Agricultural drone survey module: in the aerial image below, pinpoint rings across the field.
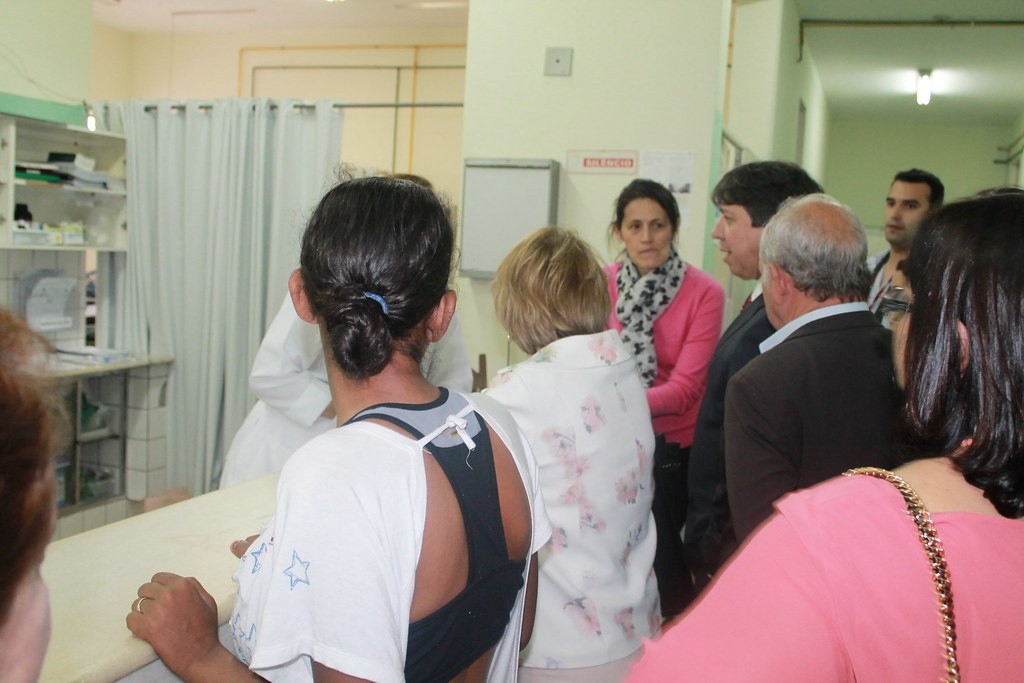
[137,598,145,612]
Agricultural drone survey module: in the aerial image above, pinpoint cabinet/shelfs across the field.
[0,112,129,251]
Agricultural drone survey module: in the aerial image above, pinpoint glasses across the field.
[880,286,914,330]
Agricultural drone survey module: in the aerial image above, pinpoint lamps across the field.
[915,67,932,105]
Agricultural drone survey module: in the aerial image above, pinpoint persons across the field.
[482,226,663,683]
[221,175,473,488]
[127,176,552,683]
[0,311,63,683]
[722,195,934,543]
[623,190,1024,683]
[599,178,726,609]
[683,161,826,597]
[866,168,945,390]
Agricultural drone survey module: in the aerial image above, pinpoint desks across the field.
[27,345,177,504]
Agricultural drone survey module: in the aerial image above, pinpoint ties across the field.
[739,291,752,314]
